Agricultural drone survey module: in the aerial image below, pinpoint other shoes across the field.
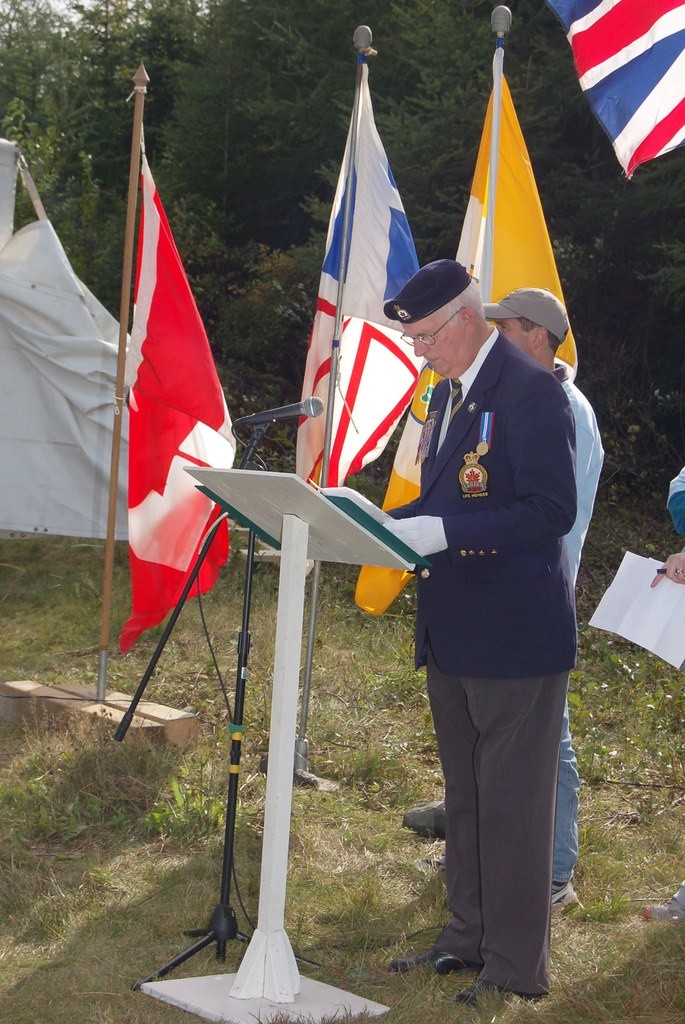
[551,886,577,913]
[647,904,672,918]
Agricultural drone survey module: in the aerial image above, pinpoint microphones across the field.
[231,396,324,428]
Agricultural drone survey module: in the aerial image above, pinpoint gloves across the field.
[383,515,448,557]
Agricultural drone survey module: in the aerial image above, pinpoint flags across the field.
[355,47,576,618]
[295,71,429,487]
[121,156,236,652]
[543,0,685,178]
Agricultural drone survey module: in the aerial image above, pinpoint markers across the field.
[656,569,678,574]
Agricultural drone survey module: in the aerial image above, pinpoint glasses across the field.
[400,307,465,346]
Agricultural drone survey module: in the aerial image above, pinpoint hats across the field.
[383,259,473,323]
[483,287,569,344]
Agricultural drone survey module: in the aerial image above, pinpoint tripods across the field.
[114,425,325,993]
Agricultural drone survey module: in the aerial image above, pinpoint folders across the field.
[195,484,433,568]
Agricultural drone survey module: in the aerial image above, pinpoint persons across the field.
[641,463,685,922]
[403,288,605,914]
[379,258,576,1007]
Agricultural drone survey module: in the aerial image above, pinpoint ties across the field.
[449,378,462,424]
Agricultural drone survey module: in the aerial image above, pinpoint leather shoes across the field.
[389,950,467,976]
[456,980,539,1006]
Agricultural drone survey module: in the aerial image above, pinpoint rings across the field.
[677,569,683,574]
